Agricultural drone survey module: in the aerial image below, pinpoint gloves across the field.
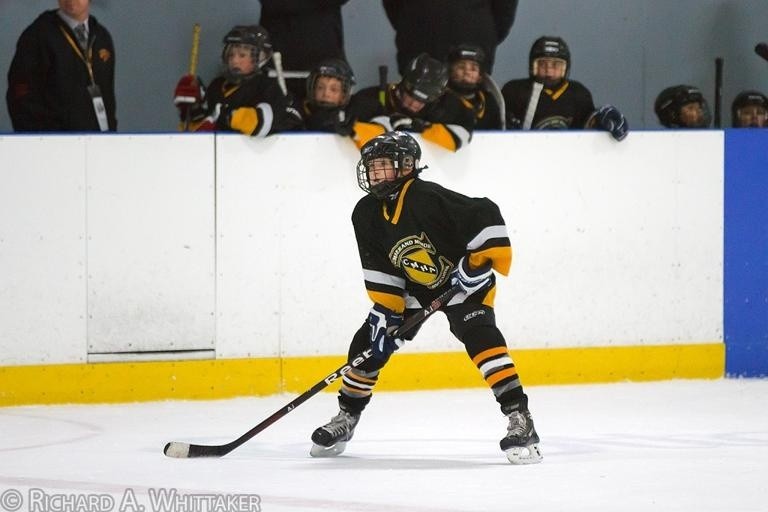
[366,302,403,361]
[175,74,233,128]
[595,106,628,141]
[450,256,492,295]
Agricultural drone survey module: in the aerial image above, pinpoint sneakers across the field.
[312,408,360,447]
[500,410,539,450]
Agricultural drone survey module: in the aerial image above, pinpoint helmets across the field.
[222,26,274,71]
[392,40,483,113]
[305,59,352,106]
[530,36,570,85]
[357,130,419,198]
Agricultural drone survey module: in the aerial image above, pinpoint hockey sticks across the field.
[165,288,463,458]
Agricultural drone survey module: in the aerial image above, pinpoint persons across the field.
[501,36,628,142]
[444,35,509,130]
[6,0,117,134]
[350,53,477,153]
[297,57,387,150]
[175,24,304,139]
[310,131,543,463]
